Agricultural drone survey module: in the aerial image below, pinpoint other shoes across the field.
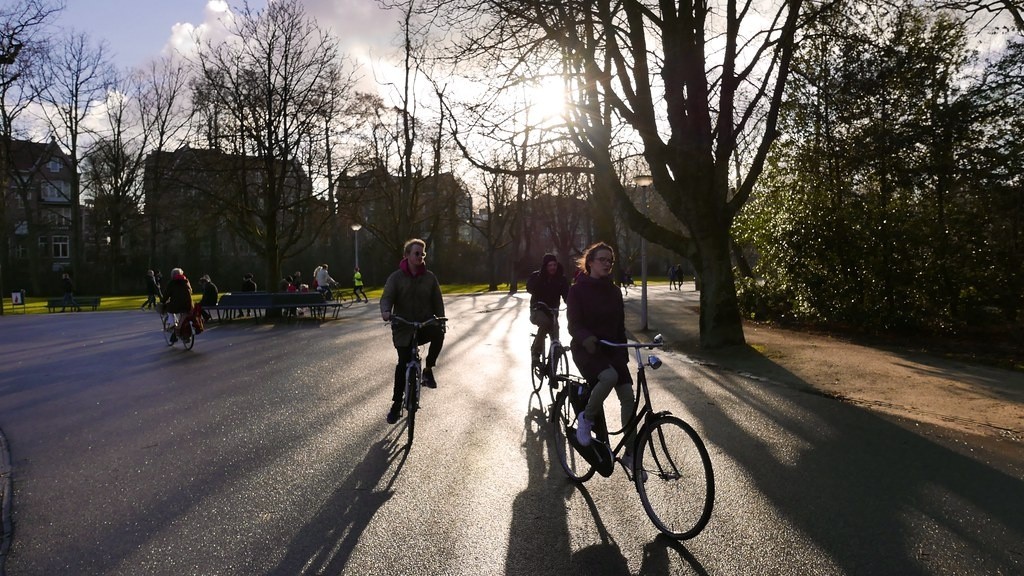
[169,323,175,329]
[532,355,540,363]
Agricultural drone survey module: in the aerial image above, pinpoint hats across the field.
[542,253,557,265]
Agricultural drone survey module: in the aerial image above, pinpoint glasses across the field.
[592,257,615,267]
[415,252,425,257]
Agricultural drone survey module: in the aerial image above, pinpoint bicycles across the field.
[551,333,715,540]
[384,314,448,444]
[530,302,571,405]
[315,282,353,308]
[155,300,195,350]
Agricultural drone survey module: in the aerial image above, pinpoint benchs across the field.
[44,296,100,313]
[202,291,343,325]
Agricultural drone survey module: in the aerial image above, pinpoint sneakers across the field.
[422,368,436,388]
[622,452,648,482]
[577,411,591,446]
[387,404,401,423]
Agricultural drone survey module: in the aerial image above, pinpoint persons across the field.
[160,268,192,343]
[312,264,338,301]
[526,253,572,389]
[620,266,637,289]
[380,240,445,424]
[236,273,256,318]
[198,275,218,322]
[353,267,369,304]
[573,267,580,283]
[141,269,161,311]
[60,273,82,312]
[567,244,648,484]
[669,264,684,291]
[279,271,309,316]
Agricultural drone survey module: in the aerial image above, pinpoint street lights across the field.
[634,175,654,330]
[351,224,362,267]
